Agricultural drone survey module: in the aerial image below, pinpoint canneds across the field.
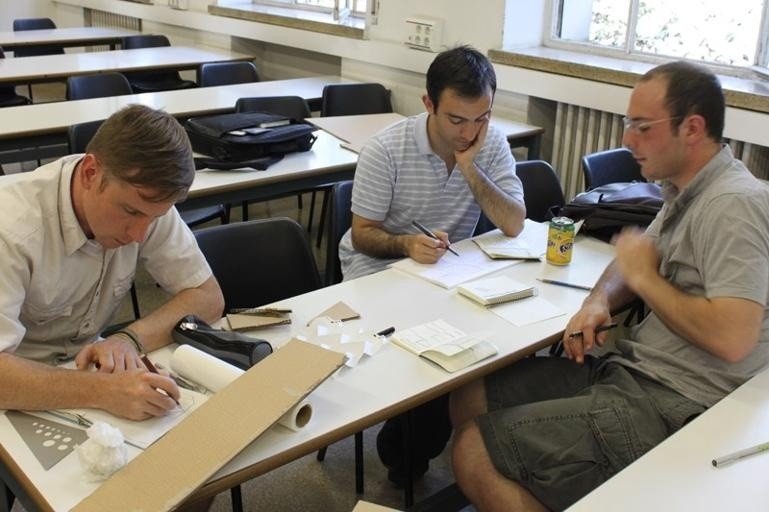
[545,216,575,265]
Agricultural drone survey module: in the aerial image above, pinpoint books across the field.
[392,319,497,373]
[458,274,536,306]
[388,240,526,290]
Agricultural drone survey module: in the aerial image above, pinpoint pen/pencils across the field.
[712,441,769,467]
[373,326,395,338]
[411,219,459,257]
[139,353,182,409]
[568,323,618,337]
[536,278,593,292]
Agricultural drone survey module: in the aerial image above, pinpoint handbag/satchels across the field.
[546,183,663,230]
[171,316,272,367]
[185,113,316,170]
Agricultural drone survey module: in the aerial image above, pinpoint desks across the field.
[562,362,769,512]
[0,219,636,512]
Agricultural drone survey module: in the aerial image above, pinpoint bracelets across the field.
[106,330,142,353]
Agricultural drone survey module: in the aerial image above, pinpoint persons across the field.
[449,60,769,511]
[0,102,226,512]
[337,42,526,488]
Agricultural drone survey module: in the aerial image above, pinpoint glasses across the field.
[623,117,677,134]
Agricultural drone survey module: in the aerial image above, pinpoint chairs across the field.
[480,161,572,356]
[584,149,644,193]
[331,178,365,285]
[190,218,365,511]
[0,16,547,241]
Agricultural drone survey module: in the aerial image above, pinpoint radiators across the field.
[90,10,140,55]
[546,100,769,205]
